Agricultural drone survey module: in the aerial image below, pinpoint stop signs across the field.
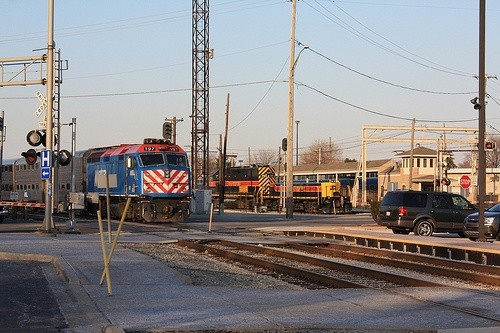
[460,176,472,189]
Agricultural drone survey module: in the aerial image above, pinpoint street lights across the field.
[294,120,301,167]
[284,45,311,218]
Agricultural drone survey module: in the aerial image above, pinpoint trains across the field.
[0,142,196,223]
[192,164,353,214]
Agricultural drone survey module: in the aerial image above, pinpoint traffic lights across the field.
[432,179,440,186]
[485,141,494,149]
[20,149,39,164]
[440,179,450,185]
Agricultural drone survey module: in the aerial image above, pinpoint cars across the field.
[461,202,500,242]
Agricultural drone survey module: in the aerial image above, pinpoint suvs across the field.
[376,188,479,237]
